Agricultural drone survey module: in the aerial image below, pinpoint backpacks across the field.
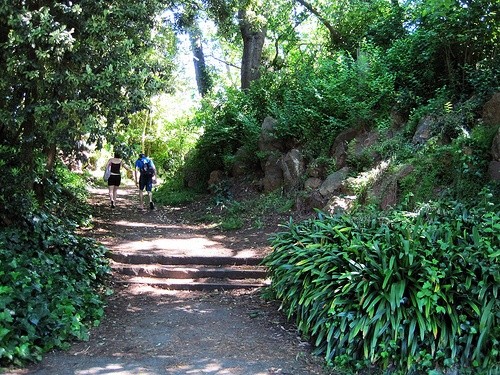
[139,158,154,177]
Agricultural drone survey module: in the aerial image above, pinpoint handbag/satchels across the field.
[104,158,112,182]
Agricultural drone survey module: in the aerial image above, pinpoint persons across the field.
[134,154,157,209]
[106,151,132,208]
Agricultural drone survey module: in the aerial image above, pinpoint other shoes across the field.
[111,201,115,208]
[150,202,154,209]
